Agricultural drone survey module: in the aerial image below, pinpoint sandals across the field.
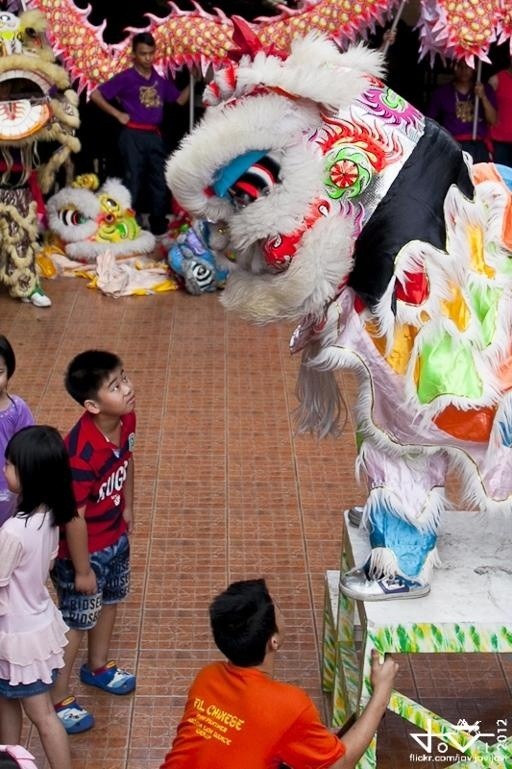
[77,658,138,696]
[54,694,95,736]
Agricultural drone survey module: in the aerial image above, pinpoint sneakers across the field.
[347,505,368,528]
[337,562,433,602]
[19,287,53,307]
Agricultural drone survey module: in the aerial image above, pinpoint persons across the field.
[43,349,139,737]
[154,577,401,769]
[1,333,36,528]
[0,79,53,309]
[350,24,398,55]
[429,54,497,165]
[1,422,83,768]
[484,51,512,167]
[331,438,447,602]
[90,31,213,240]
[0,740,38,768]
